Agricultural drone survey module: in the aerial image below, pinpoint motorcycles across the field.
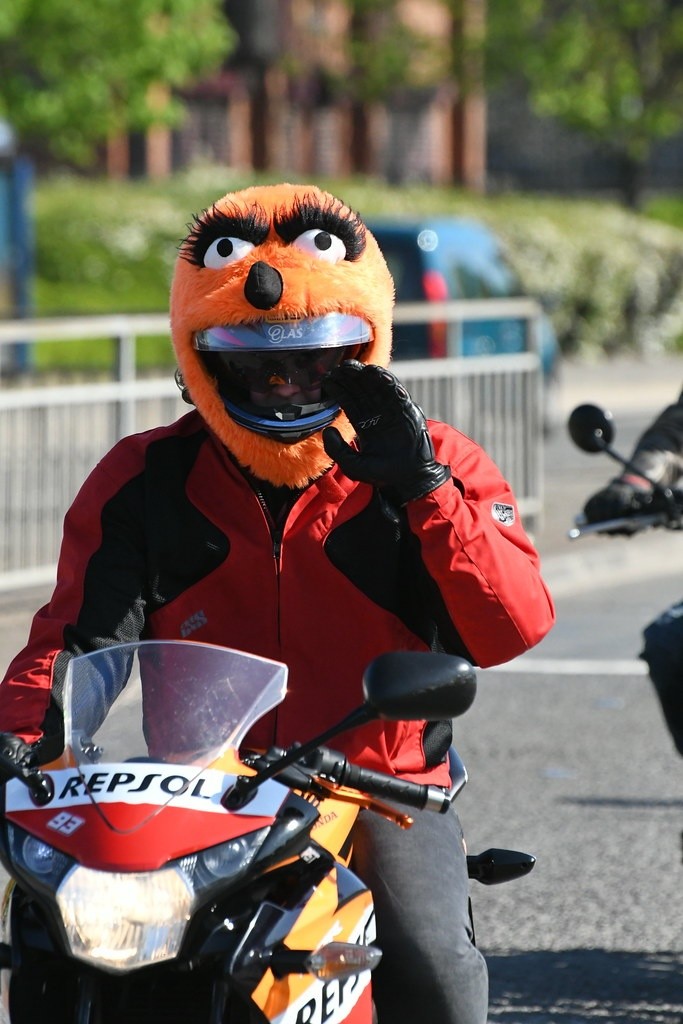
[0,640,537,1024]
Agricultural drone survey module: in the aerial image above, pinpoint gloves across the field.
[583,478,657,538]
[323,360,451,510]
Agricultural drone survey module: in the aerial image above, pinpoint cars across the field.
[362,215,558,382]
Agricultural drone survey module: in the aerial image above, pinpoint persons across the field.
[1,182,558,1023]
[580,382,683,754]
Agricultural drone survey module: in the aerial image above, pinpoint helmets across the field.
[168,185,397,493]
